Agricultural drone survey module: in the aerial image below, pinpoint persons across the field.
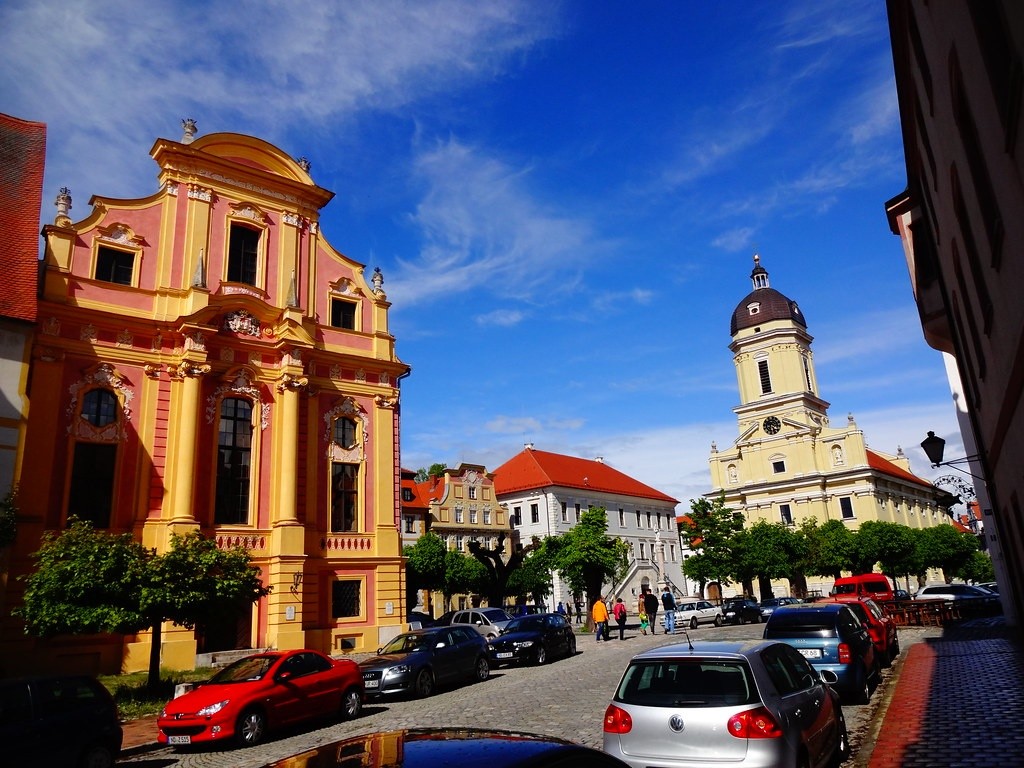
[557,601,583,624]
[592,587,678,643]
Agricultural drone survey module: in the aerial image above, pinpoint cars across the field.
[659,601,723,630]
[488,613,576,666]
[719,597,762,625]
[257,726,634,768]
[762,604,884,706]
[157,649,365,747]
[814,595,902,662]
[602,639,850,768]
[0,673,123,768]
[357,624,490,700]
[892,581,999,609]
[407,605,548,658]
[759,597,801,623]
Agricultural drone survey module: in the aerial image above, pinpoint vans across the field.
[830,574,895,604]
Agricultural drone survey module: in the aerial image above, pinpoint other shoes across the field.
[640,629,647,636]
[664,629,668,634]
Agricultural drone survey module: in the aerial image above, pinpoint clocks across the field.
[763,416,782,436]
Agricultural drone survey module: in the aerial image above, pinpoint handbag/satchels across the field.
[619,610,627,621]
[579,607,581,611]
[562,610,566,616]
[639,612,648,621]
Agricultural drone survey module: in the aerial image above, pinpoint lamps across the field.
[290,571,303,595]
[975,527,999,551]
[920,431,992,485]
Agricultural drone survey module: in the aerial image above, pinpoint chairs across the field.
[646,662,737,695]
